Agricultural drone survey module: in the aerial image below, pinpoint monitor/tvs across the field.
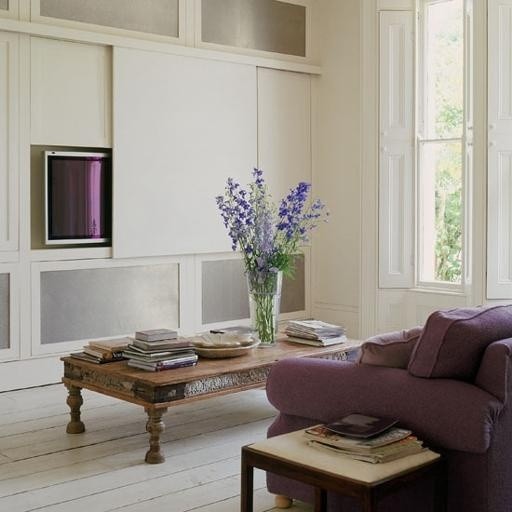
[45,151,112,245]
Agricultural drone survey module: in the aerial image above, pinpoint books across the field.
[210,327,259,335]
[302,413,429,464]
[70,329,198,372]
[280,320,347,347]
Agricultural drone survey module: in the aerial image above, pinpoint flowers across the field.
[214,163,331,341]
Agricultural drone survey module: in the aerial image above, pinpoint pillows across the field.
[356,326,423,369]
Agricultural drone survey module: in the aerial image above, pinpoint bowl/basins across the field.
[189,334,261,358]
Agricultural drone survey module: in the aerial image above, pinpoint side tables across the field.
[239,422,441,511]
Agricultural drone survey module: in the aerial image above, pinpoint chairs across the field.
[264,302,511,511]
